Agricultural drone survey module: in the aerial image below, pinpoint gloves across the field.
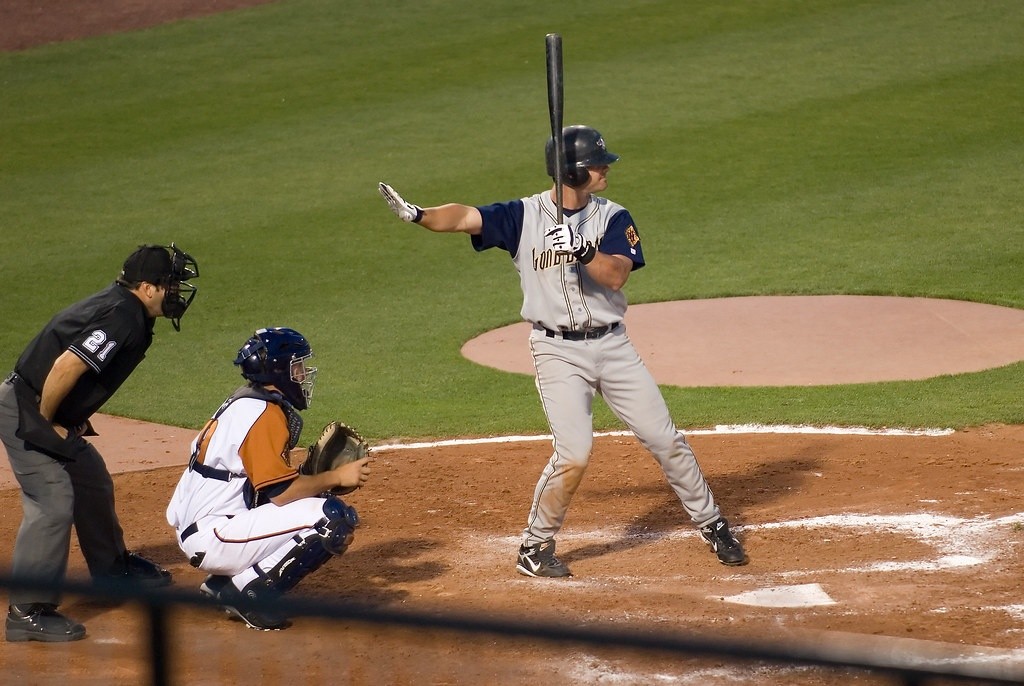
[544,224,596,266]
[190,551,205,568]
[379,182,424,223]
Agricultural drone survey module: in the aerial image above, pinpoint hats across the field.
[121,246,192,283]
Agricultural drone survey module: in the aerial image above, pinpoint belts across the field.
[543,322,619,341]
[181,515,234,543]
[7,372,18,384]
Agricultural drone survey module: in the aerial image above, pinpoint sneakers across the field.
[200,573,231,597]
[699,517,746,565]
[92,553,172,586]
[5,602,86,642]
[217,580,287,631]
[516,539,573,577]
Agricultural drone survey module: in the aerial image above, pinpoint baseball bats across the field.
[545,32,568,256]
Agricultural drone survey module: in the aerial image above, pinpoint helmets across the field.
[545,125,619,186]
[234,328,317,411]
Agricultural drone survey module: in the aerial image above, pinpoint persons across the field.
[377,124,745,578]
[0,243,201,642]
[165,326,372,631]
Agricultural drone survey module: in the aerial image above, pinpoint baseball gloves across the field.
[298,421,371,496]
